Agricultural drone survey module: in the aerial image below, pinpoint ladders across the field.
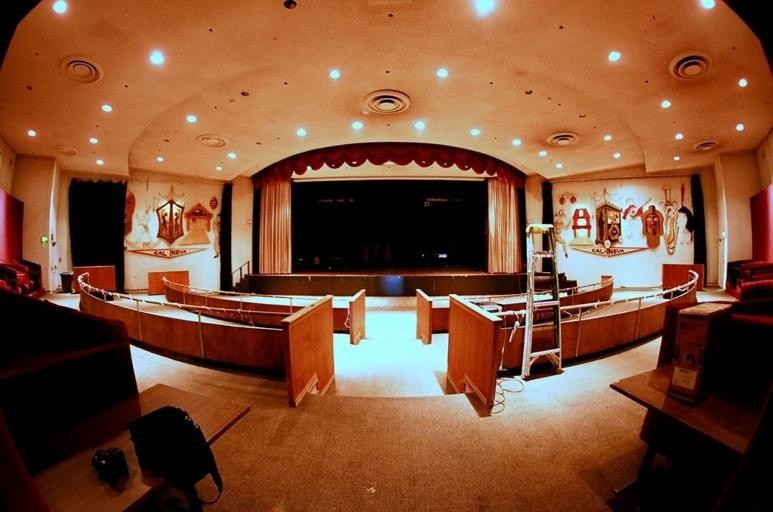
[521,224,565,381]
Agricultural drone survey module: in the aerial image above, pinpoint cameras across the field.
[90,447,128,474]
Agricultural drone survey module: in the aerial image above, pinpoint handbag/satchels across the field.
[127,405,223,505]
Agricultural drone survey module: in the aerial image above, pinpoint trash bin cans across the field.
[59,272,74,293]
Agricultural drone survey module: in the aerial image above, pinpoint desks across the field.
[604,367,765,512]
[5,385,251,512]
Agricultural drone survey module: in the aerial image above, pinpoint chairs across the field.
[0,256,46,297]
[722,259,773,302]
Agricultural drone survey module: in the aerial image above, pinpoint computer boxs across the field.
[665,302,733,405]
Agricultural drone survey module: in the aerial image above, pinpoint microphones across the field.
[508,320,521,343]
